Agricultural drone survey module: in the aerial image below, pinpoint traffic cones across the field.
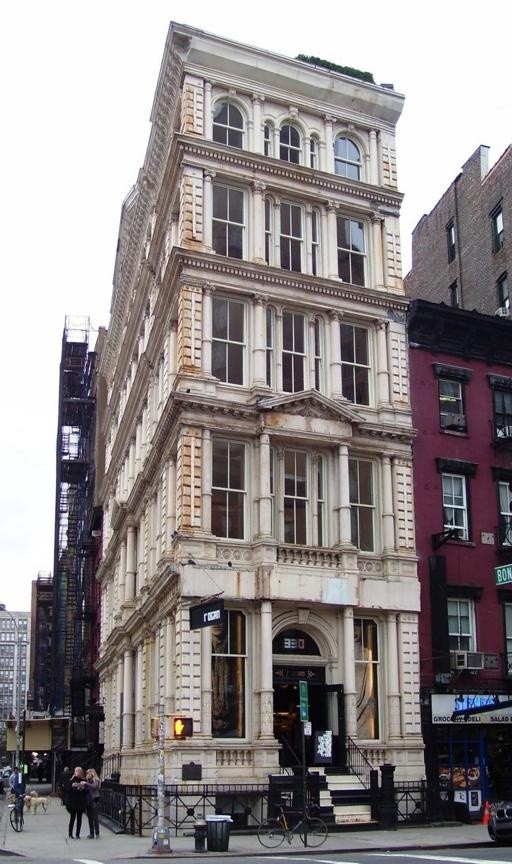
[481,799,490,826]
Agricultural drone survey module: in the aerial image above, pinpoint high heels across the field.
[69,832,80,838]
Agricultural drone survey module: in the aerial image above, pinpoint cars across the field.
[488,796,511,847]
[0,767,13,788]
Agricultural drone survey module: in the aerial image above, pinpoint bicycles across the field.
[257,800,329,849]
[10,792,27,832]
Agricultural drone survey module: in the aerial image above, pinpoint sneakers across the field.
[87,834,101,839]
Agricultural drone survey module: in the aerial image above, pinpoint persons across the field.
[80,768,101,839]
[65,766,87,840]
[8,766,25,802]
[36,763,44,783]
[58,766,70,806]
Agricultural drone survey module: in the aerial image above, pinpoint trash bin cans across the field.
[205,815,233,852]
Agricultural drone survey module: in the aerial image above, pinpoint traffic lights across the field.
[174,717,193,737]
